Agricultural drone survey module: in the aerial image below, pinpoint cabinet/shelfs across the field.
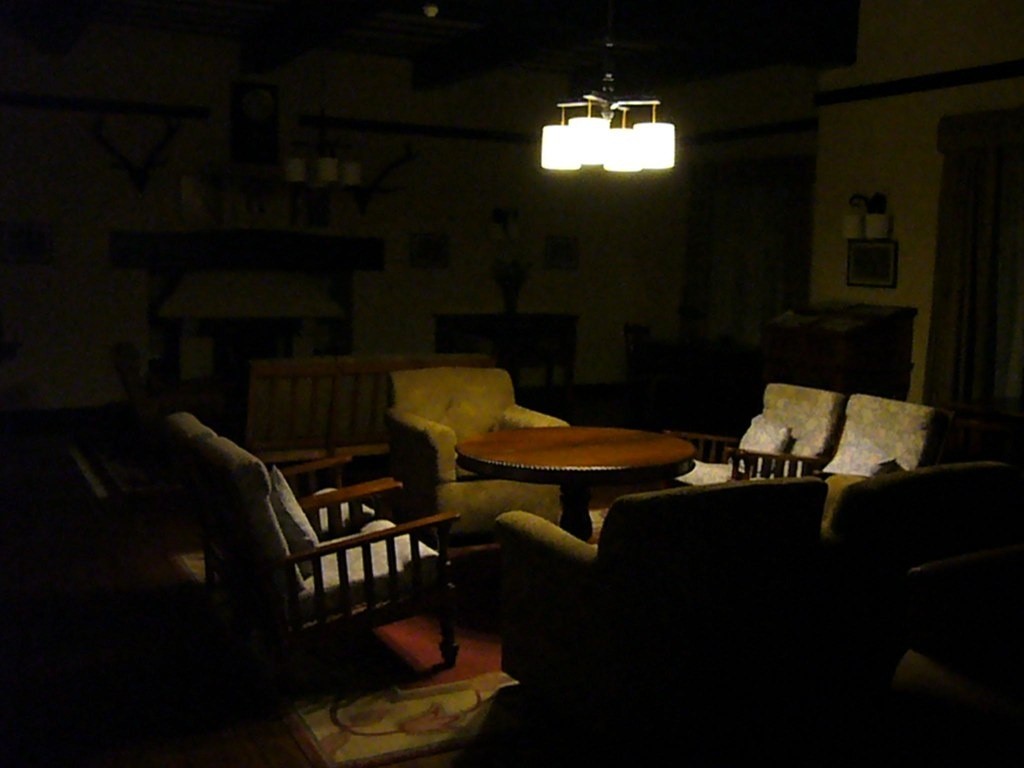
[436,312,579,421]
[762,301,919,401]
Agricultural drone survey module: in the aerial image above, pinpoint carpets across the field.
[161,508,613,767]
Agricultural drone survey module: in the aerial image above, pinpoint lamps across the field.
[533,0,688,188]
[281,52,365,188]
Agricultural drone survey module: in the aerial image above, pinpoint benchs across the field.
[241,354,496,491]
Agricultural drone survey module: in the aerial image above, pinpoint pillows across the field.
[728,415,791,473]
[822,434,894,478]
[269,464,319,576]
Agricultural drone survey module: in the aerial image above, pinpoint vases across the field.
[495,263,526,311]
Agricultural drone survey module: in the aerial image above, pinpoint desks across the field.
[453,425,697,543]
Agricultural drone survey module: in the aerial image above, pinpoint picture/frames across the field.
[845,236,899,289]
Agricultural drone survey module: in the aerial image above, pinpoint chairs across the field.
[165,411,462,719]
[662,381,955,487]
[113,343,227,433]
[384,365,570,555]
[494,458,1024,768]
